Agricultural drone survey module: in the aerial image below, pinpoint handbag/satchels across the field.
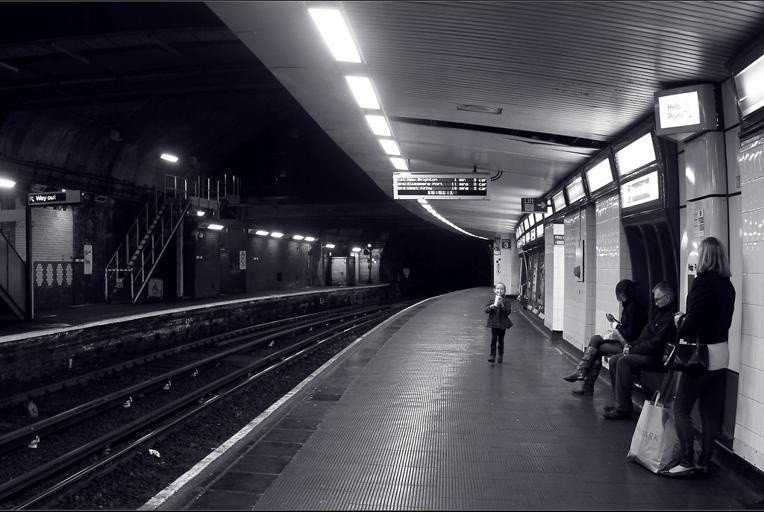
[663,313,709,372]
[626,390,682,475]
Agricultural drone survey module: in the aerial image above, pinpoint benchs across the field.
[603,312,687,406]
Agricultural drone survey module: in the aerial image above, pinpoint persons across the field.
[484,281,514,365]
[601,280,679,420]
[658,237,736,478]
[563,279,650,399]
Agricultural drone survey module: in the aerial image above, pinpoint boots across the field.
[564,346,602,395]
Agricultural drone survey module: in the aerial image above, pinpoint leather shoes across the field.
[659,469,696,476]
[603,404,634,419]
